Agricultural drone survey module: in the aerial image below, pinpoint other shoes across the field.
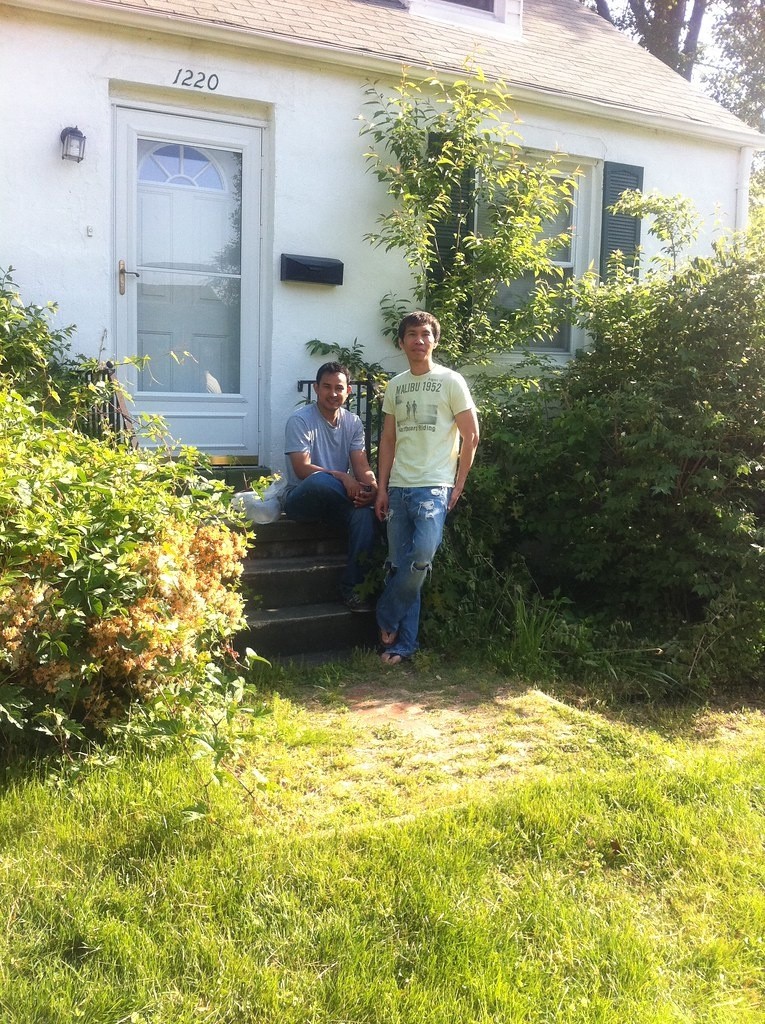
[349,591,373,612]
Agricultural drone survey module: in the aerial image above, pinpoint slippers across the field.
[381,653,404,665]
[379,625,396,647]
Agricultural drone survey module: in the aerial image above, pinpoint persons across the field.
[373,311,479,666]
[283,362,386,613]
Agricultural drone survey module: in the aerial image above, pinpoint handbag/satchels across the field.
[231,472,288,524]
